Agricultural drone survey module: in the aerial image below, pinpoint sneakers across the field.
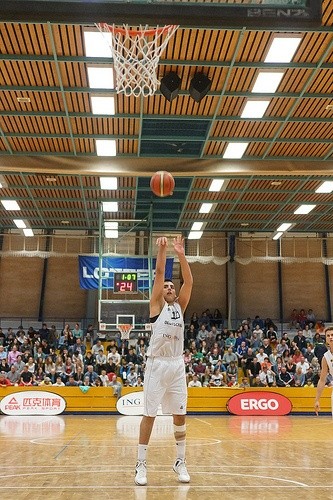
[173,458,190,482]
[135,460,147,486]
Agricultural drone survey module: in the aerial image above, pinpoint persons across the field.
[314,326,333,419]
[0,322,148,388]
[185,308,333,387]
[134,235,193,486]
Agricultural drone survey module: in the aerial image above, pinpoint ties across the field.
[90,372,92,384]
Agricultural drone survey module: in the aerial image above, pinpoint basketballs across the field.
[150,171,176,198]
